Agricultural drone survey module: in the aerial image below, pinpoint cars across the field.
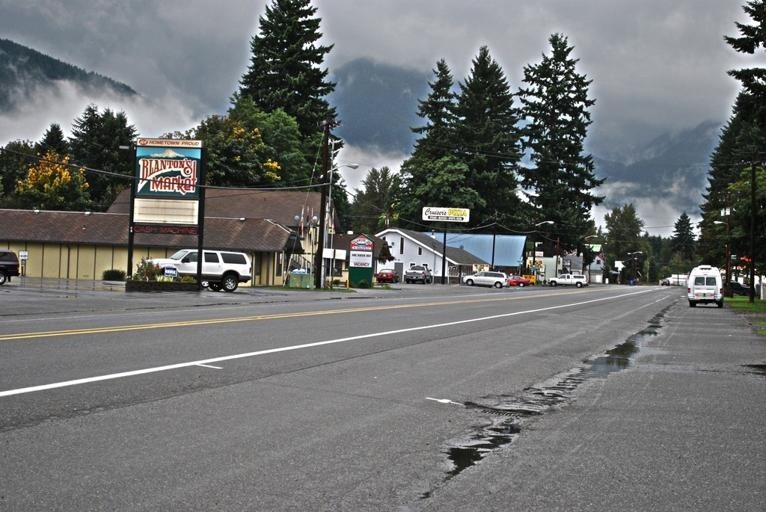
[0,251,20,285]
[463,271,530,288]
[140,249,252,292]
[661,280,670,286]
[376,269,400,282]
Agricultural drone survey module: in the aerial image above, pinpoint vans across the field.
[688,265,723,307]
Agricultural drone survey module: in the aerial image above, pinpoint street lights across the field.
[714,221,730,286]
[588,235,598,284]
[533,221,554,275]
[314,118,359,288]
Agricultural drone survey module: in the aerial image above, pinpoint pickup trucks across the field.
[548,274,587,288]
[405,266,432,284]
